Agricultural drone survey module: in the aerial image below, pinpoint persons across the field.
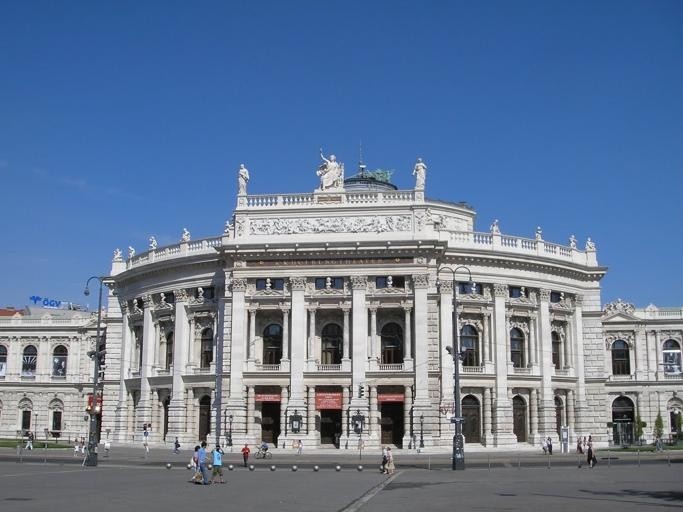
[186,442,225,484]
[295,440,302,455]
[173,436,180,455]
[318,148,343,187]
[542,435,597,468]
[238,162,248,196]
[240,442,250,468]
[411,157,427,189]
[24,432,33,450]
[80,436,87,456]
[385,447,394,474]
[72,438,79,456]
[256,441,268,457]
[380,447,388,475]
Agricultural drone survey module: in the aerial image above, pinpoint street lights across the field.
[433,264,477,470]
[417,413,423,449]
[83,274,121,468]
[227,413,233,446]
[33,411,38,438]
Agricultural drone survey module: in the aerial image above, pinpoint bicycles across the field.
[255,446,272,460]
